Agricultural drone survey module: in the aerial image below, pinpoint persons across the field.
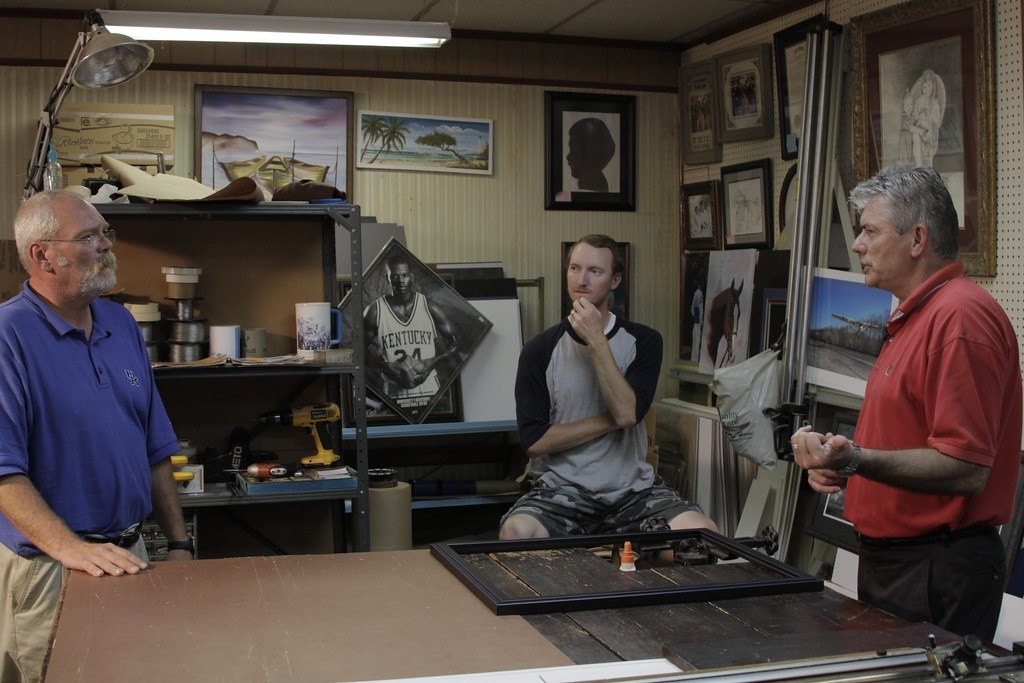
[690,278,704,365]
[690,74,757,131]
[790,160,1024,645]
[499,235,719,560]
[0,188,192,683]
[692,200,710,234]
[362,256,458,419]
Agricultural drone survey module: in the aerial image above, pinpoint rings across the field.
[792,444,798,449]
[570,311,576,317]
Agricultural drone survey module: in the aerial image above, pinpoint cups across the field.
[245,328,267,357]
[296,302,342,361]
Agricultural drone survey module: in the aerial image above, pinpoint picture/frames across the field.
[543,89,638,211]
[797,266,901,399]
[848,0,997,280]
[678,11,826,165]
[338,236,493,424]
[357,109,494,175]
[558,239,630,324]
[807,407,866,557]
[678,157,792,375]
[193,83,354,203]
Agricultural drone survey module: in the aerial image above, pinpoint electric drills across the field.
[247,403,340,466]
[222,463,292,478]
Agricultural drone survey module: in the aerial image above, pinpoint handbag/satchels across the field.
[707,322,787,473]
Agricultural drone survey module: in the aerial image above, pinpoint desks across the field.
[39,540,1024,683]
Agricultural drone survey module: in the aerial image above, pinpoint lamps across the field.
[97,7,451,48]
[21,10,155,206]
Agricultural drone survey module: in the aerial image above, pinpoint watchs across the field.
[834,444,861,477]
[167,537,196,554]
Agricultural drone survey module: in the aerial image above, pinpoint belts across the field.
[82,519,145,548]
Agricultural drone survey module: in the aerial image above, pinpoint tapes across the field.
[168,282,196,299]
[166,274,198,283]
[124,302,158,313]
[131,312,161,321]
[161,265,202,274]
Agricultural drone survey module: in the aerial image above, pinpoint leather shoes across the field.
[854,519,999,549]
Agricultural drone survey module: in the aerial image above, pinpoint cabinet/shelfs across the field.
[21,206,372,556]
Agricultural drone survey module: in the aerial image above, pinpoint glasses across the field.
[40,229,117,249]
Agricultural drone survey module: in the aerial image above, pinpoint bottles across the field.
[619,541,636,571]
